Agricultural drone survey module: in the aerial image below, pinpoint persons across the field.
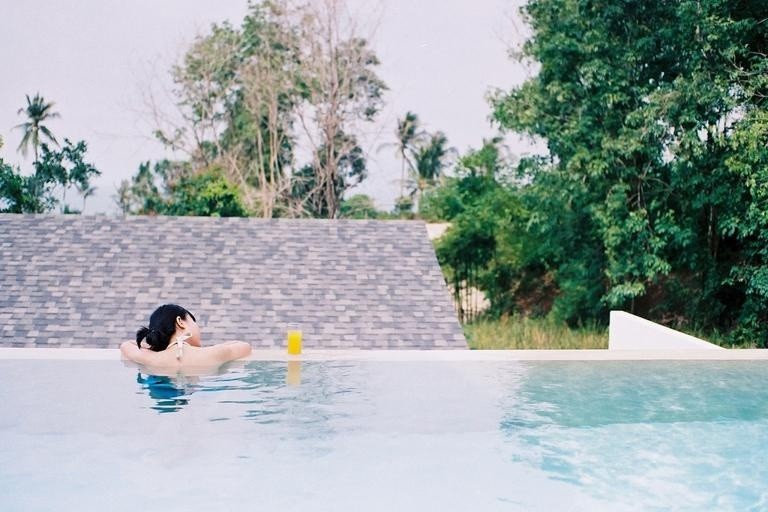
[121,303,251,379]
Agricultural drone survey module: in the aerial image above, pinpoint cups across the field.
[286,322,303,356]
